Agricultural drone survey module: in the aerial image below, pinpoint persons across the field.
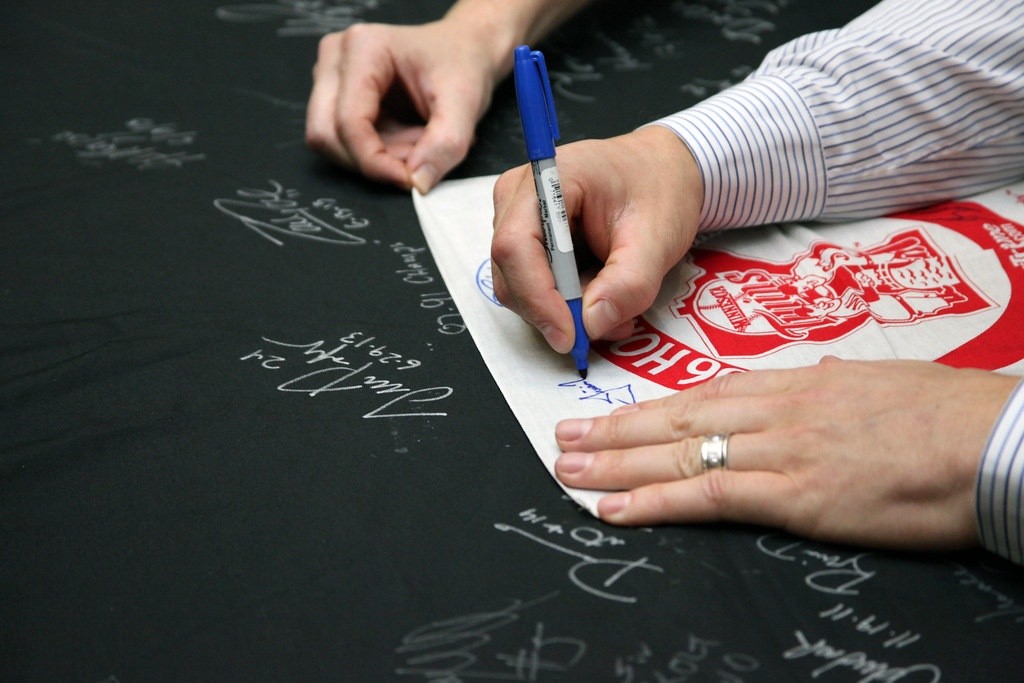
[305,0,585,195]
[491,0,1024,566]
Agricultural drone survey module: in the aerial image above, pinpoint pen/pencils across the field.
[514,44,592,381]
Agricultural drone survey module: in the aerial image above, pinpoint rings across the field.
[700,432,734,470]
